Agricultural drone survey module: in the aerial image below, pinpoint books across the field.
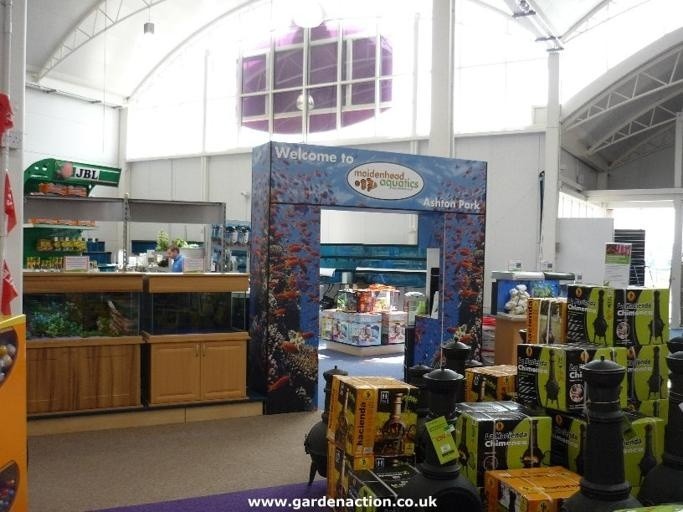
[485,465,583,512]
[328,443,415,512]
[465,365,517,401]
[630,343,670,401]
[556,412,664,483]
[454,401,552,488]
[348,468,423,510]
[526,296,568,346]
[568,286,672,345]
[516,347,628,410]
[327,374,420,456]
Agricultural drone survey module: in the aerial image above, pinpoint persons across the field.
[168,245,184,272]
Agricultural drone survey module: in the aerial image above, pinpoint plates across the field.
[146,272,250,407]
[23,270,144,418]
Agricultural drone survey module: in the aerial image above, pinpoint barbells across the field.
[273,308,287,317]
[267,375,290,394]
[447,326,458,334]
[274,291,298,300]
[458,289,478,297]
[287,244,301,253]
[302,332,313,338]
[278,341,299,356]
[461,262,480,273]
[285,260,298,267]
[459,335,474,343]
